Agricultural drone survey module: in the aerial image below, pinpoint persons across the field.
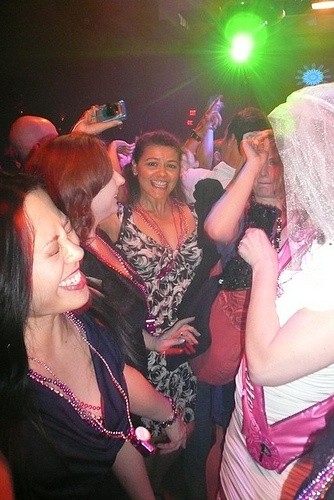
[1,171,188,500]
[92,130,205,443]
[189,104,287,487]
[3,102,60,167]
[199,105,272,171]
[17,133,203,455]
[216,81,333,500]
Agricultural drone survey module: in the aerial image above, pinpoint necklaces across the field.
[135,203,191,251]
[90,233,150,291]
[23,310,158,453]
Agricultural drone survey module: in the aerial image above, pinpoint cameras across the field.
[94,98,127,123]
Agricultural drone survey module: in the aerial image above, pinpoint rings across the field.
[180,331,183,336]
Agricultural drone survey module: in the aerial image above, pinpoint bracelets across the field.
[157,399,183,430]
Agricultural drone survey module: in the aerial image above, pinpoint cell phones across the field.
[135,441,157,456]
